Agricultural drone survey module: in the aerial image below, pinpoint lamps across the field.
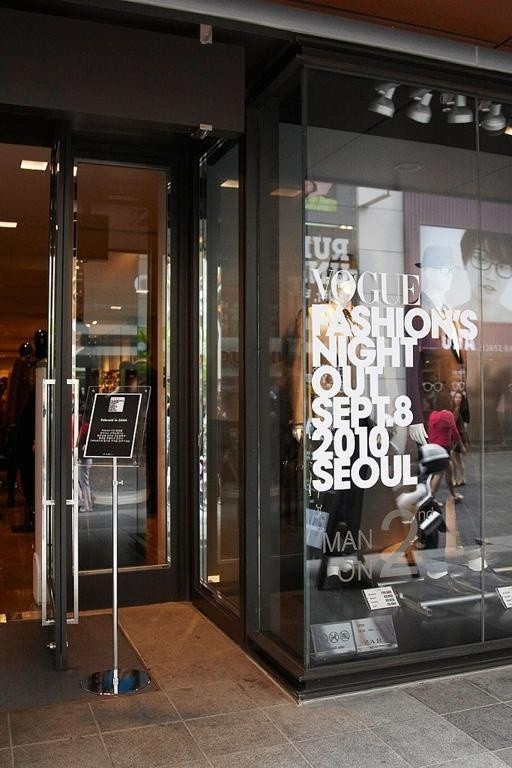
[366,80,507,137]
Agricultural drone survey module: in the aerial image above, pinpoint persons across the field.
[290,253,375,591]
[77,458,92,512]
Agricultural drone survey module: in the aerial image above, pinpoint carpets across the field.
[0,612,160,722]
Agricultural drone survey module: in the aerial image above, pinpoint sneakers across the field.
[434,476,467,507]
